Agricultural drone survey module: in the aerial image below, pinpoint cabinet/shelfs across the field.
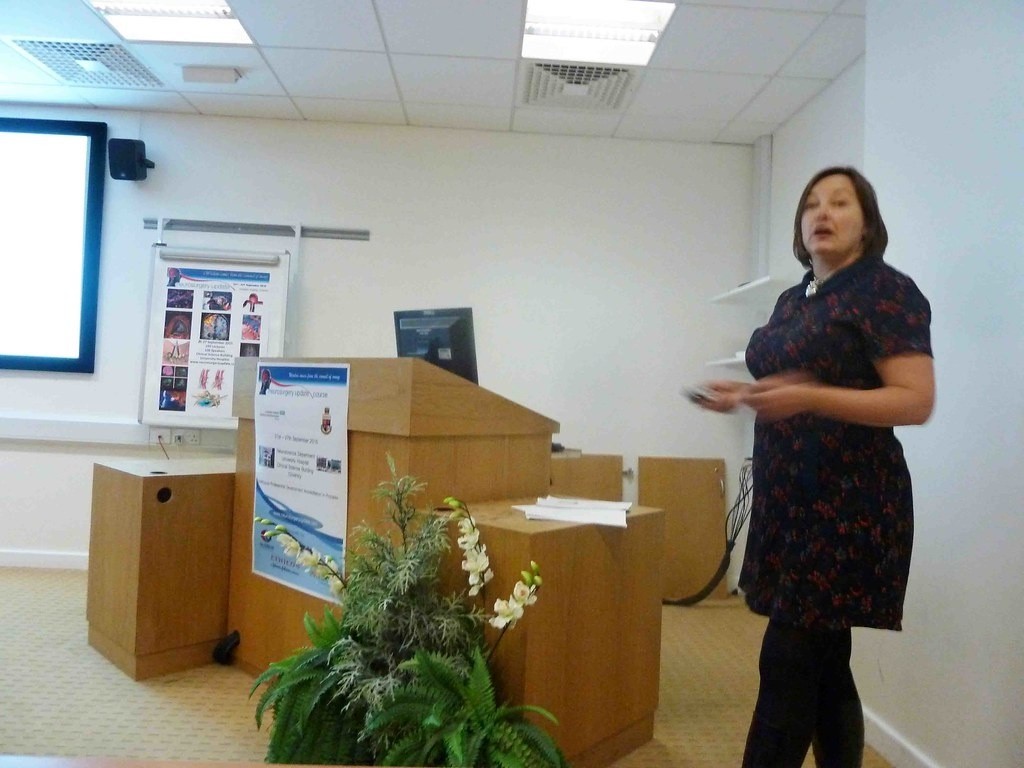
[707,276,786,373]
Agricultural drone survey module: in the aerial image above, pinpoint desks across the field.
[413,493,664,768]
[86,459,237,682]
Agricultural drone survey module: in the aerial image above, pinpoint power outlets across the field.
[149,427,171,445]
[172,429,200,445]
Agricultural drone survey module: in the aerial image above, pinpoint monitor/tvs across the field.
[394,306,480,388]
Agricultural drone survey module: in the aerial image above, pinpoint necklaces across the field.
[804,259,845,296]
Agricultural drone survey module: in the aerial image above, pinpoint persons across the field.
[696,166,936,768]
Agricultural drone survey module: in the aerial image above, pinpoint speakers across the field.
[108,139,148,181]
